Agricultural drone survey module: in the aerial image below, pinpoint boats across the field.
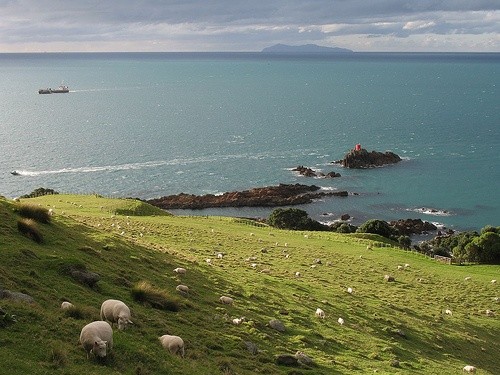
[38,87,69,94]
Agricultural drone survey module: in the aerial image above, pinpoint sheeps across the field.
[38,198,500,375]
